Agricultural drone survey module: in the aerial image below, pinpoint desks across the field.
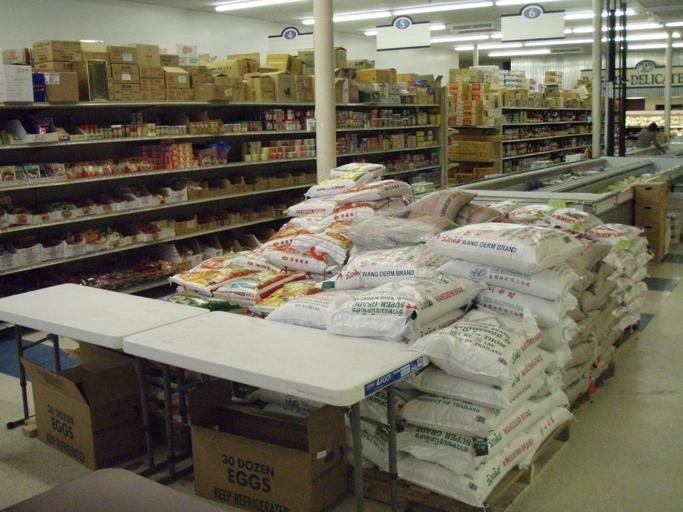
[1,466,244,510]
[0,281,210,485]
[123,308,427,507]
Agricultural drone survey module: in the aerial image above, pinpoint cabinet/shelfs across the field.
[0,99,619,295]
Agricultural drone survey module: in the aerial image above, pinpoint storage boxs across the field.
[0,40,398,104]
[181,381,353,511]
[15,343,143,471]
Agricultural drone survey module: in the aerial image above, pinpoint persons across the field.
[635,122,666,154]
